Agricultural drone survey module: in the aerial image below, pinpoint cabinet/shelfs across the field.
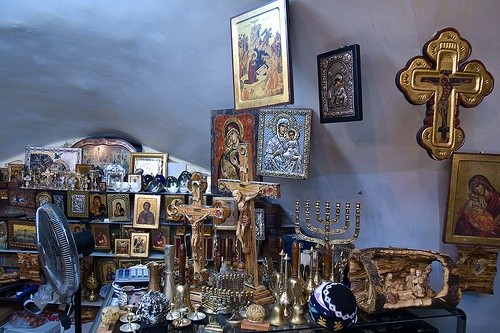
[0,189,276,309]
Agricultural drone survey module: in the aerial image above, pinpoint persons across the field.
[225,185,264,255]
[183,210,210,265]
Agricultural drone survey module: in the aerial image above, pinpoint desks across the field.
[88,279,467,333]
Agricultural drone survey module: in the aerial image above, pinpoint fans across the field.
[36,204,96,333]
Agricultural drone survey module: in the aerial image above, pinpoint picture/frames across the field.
[210,0,313,193]
[129,152,167,184]
[442,152,500,244]
[316,43,363,124]
[0,146,95,186]
[0,189,265,333]
